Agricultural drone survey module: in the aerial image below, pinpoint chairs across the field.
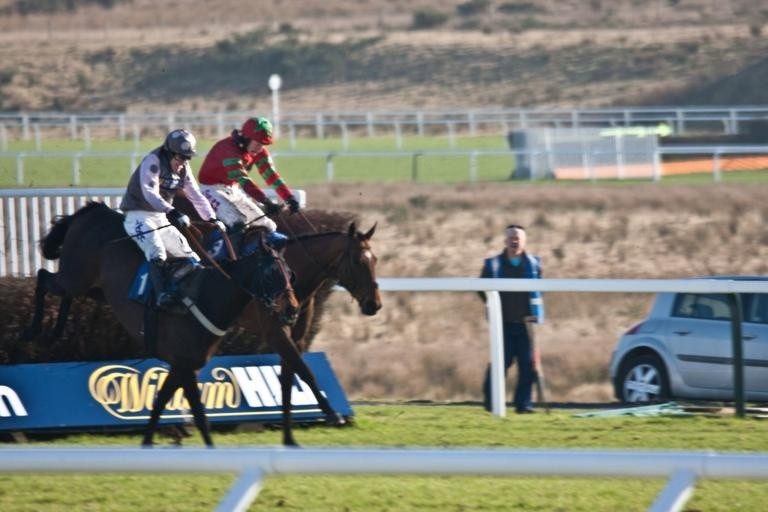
[688,303,714,319]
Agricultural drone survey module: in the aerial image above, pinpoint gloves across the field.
[263,197,281,216]
[168,210,190,228]
[210,219,227,232]
[287,197,299,211]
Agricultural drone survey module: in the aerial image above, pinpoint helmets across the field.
[242,117,273,145]
[163,129,198,157]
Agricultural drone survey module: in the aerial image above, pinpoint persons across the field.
[119,127,230,309]
[476,221,547,415]
[196,115,302,234]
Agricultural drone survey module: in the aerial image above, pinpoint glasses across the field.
[166,130,191,162]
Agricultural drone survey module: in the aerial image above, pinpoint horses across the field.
[18,200,303,447]
[189,222,382,446]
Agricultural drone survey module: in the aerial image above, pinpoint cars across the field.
[606,273,767,407]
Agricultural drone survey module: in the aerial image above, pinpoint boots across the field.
[150,258,173,312]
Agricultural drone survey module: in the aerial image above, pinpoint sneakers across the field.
[517,407,533,413]
